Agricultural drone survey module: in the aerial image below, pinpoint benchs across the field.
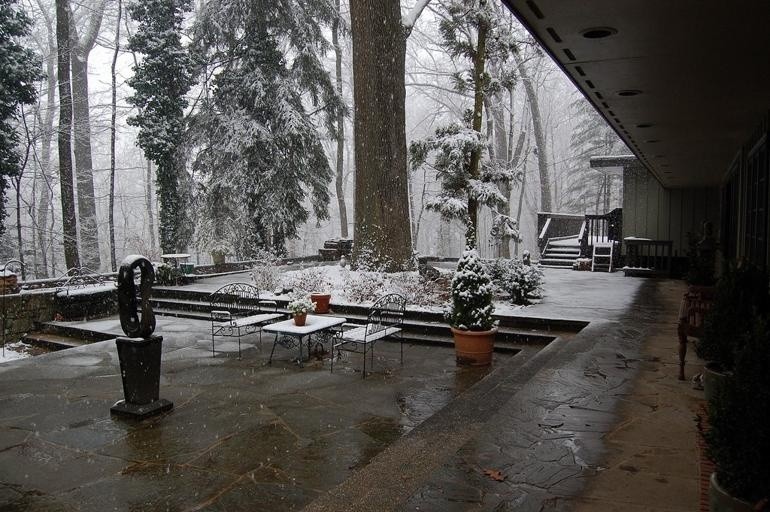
[678,285,717,381]
[330,292,408,380]
[208,281,285,361]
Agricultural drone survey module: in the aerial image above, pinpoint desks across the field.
[261,315,348,370]
[161,253,190,288]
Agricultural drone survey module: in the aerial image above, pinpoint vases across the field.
[311,292,331,314]
[441,244,500,367]
[294,314,306,326]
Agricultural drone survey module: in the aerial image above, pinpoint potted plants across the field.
[697,256,770,512]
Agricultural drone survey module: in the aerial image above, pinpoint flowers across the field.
[288,300,310,314]
